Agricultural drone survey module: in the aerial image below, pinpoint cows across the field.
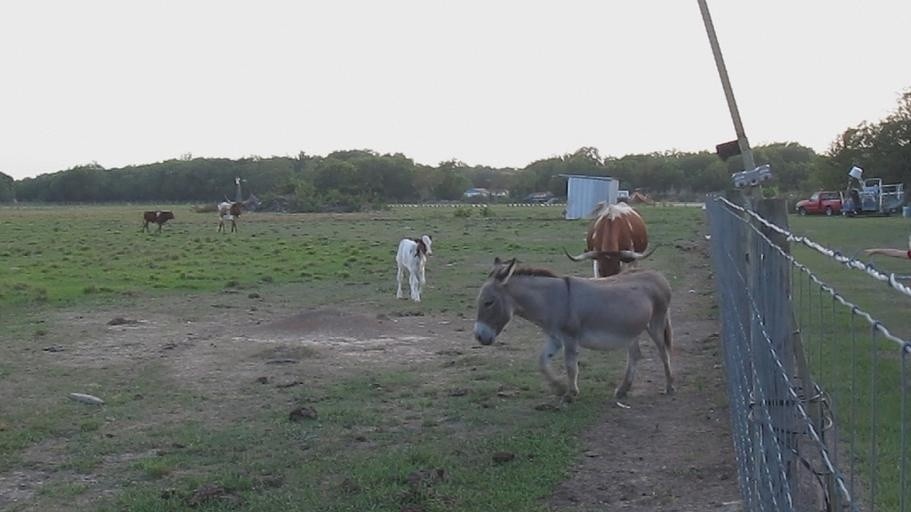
[216,201,242,234]
[141,210,175,233]
[561,201,663,279]
[395,235,433,303]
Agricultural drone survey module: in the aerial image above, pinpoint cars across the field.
[521,191,559,204]
[462,187,512,202]
[795,190,843,216]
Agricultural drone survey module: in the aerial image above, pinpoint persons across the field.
[216,199,231,233]
[863,235,911,260]
[224,193,241,233]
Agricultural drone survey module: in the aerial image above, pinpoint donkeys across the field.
[472,257,675,408]
